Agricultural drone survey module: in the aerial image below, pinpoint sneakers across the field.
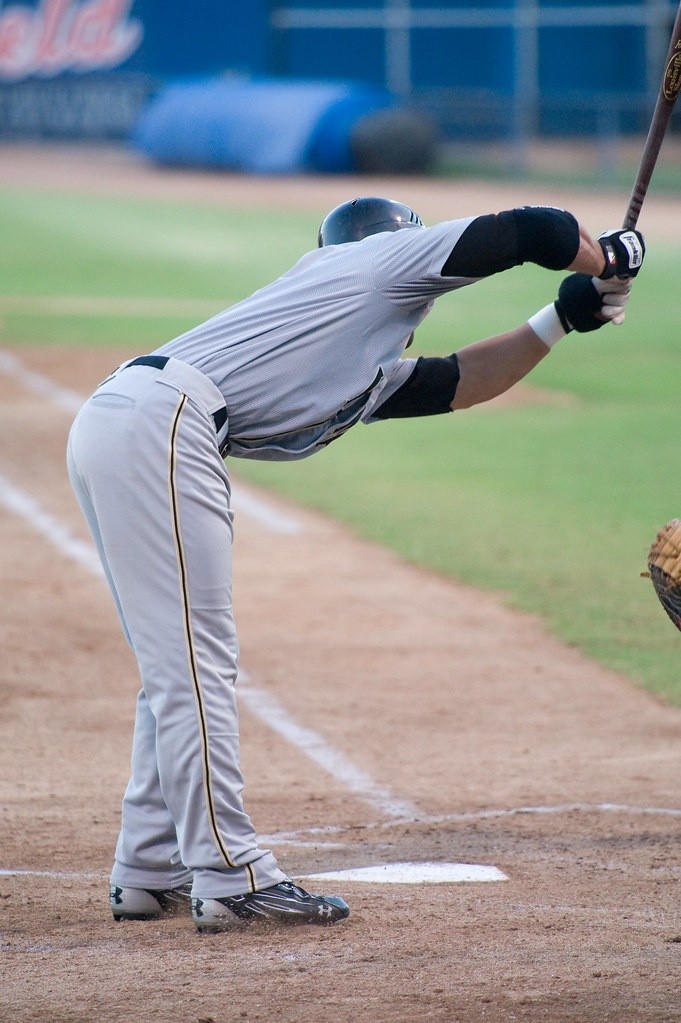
[110,882,352,933]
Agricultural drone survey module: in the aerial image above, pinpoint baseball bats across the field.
[618,0,681,232]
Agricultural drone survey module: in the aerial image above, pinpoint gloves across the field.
[559,227,647,333]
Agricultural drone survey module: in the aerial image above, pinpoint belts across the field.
[123,356,233,461]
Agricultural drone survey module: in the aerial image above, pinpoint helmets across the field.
[319,197,425,244]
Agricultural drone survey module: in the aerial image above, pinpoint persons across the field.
[67,197,646,932]
